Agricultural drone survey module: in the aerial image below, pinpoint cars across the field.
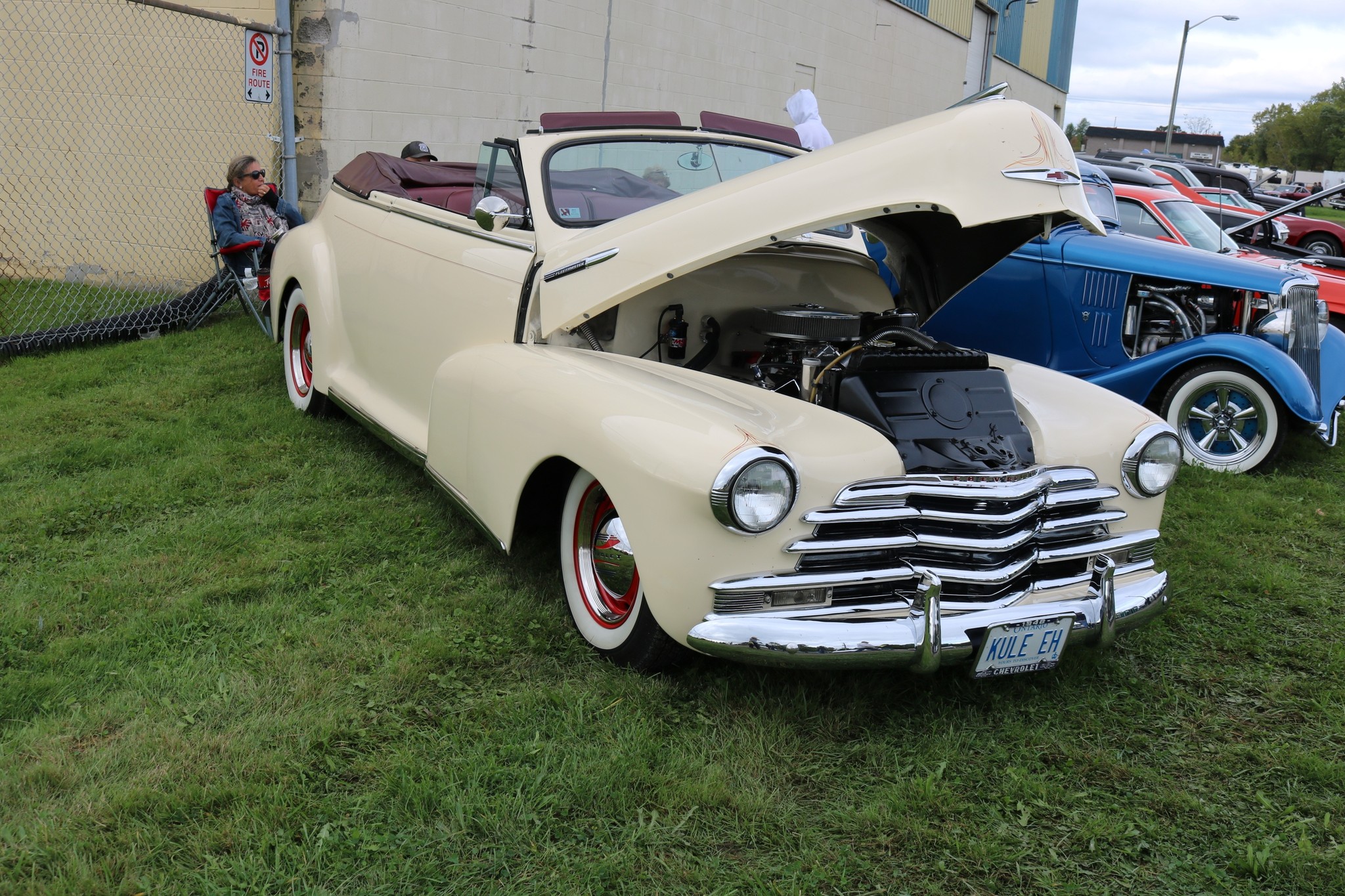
[858,149,1344,475]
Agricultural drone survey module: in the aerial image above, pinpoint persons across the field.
[642,166,671,190]
[769,88,835,167]
[1310,182,1320,206]
[212,155,306,279]
[401,141,438,163]
[1316,182,1324,207]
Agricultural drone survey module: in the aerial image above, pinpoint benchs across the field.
[405,185,668,225]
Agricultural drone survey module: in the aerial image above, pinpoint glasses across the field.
[238,169,266,180]
[655,171,667,176]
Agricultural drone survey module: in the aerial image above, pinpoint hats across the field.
[401,141,438,161]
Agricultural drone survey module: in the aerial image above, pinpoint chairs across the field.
[185,182,279,338]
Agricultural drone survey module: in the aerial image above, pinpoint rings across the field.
[263,192,266,195]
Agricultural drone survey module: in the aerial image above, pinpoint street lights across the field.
[1162,12,1241,154]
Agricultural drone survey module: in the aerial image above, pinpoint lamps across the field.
[1004,0,1038,17]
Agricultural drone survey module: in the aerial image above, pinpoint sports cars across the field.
[267,107,1186,679]
